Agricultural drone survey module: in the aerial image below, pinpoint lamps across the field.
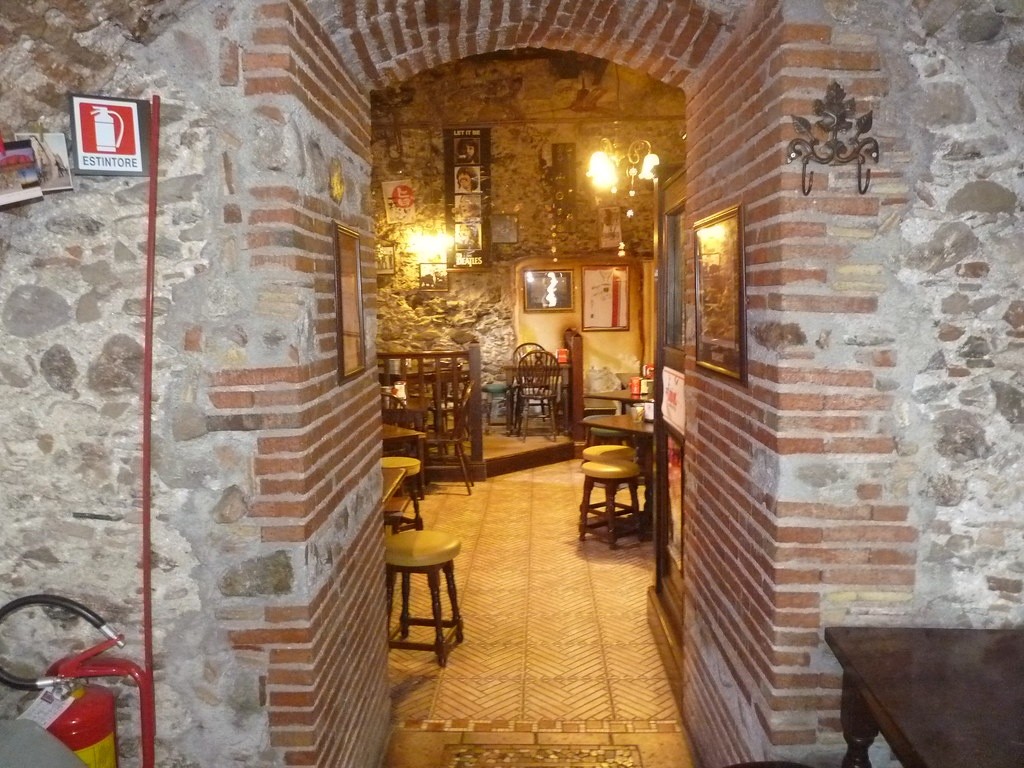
[585,64,661,194]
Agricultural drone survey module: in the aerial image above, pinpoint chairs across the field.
[382,393,411,459]
[513,343,561,442]
[418,379,476,496]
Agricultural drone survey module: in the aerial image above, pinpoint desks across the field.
[504,364,571,437]
[580,413,653,542]
[822,624,1024,768]
[382,423,427,490]
[383,467,409,509]
[583,372,654,419]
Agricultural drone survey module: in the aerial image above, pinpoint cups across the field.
[389,359,400,371]
[382,387,392,400]
[631,407,643,422]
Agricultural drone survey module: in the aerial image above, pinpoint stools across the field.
[483,384,508,437]
[584,398,617,416]
[580,426,647,542]
[382,456,423,535]
[383,528,464,668]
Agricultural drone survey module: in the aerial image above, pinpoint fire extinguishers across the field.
[0,593,127,768]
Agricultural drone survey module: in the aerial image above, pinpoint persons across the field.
[459,194,481,220]
[456,167,479,192]
[456,138,479,164]
[459,224,479,250]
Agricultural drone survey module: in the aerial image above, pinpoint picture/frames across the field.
[332,219,368,387]
[419,262,449,293]
[580,264,631,332]
[522,268,576,314]
[693,202,749,385]
